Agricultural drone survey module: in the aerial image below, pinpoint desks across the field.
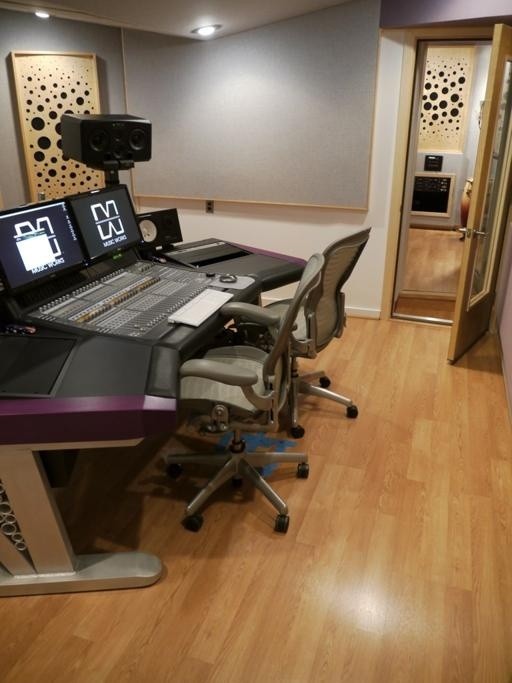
[0,243,307,597]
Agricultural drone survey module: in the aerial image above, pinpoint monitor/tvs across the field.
[0,332,82,398]
[63,183,143,267]
[0,197,88,292]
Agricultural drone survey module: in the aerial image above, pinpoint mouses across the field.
[220,272,238,285]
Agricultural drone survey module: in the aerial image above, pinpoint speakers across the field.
[62,112,153,163]
[136,208,183,252]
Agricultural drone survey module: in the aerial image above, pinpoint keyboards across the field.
[166,288,234,328]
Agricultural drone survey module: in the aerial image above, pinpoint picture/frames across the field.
[418,45,476,154]
[6,51,106,203]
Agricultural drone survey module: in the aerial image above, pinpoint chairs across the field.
[164,252,325,534]
[227,230,371,438]
